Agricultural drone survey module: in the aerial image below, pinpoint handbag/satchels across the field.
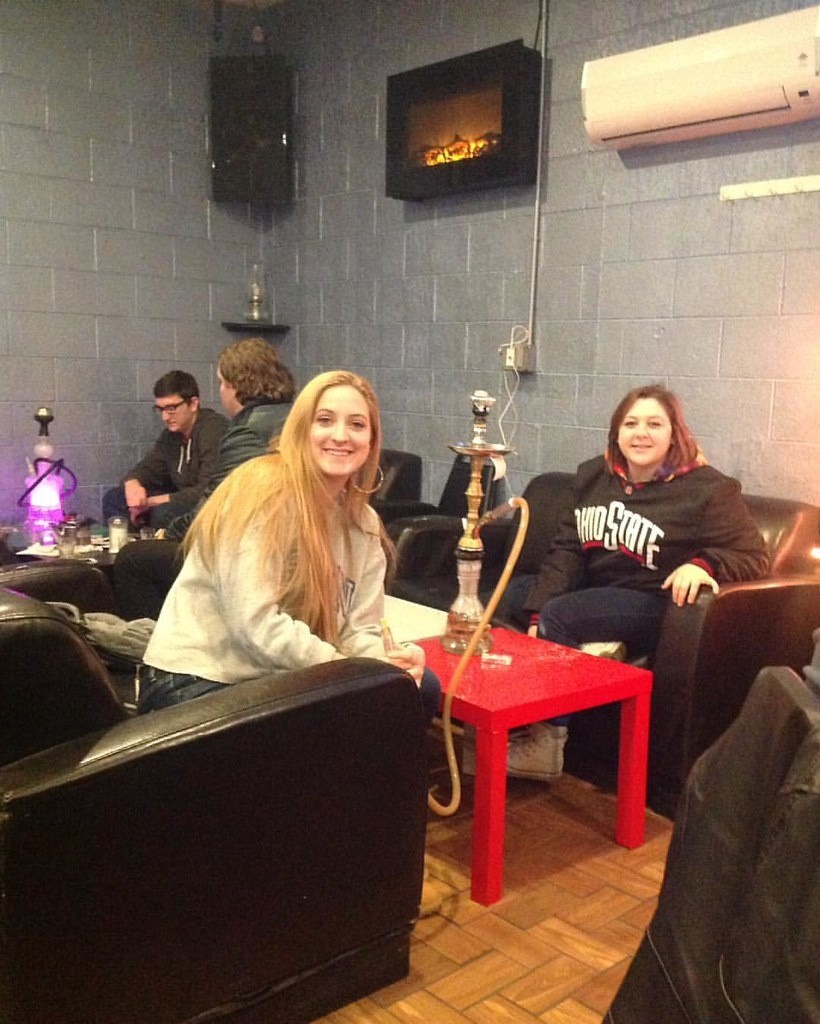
[44,601,157,658]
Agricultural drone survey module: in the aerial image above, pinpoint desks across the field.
[379,595,653,907]
[1,520,142,578]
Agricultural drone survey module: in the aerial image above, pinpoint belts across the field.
[139,666,168,694]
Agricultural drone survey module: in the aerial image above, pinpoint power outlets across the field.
[501,343,536,373]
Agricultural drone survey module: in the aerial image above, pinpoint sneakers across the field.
[579,641,627,662]
[507,722,569,782]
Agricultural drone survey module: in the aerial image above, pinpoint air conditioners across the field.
[580,3,820,150]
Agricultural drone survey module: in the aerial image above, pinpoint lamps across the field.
[440,390,516,656]
[244,261,268,324]
[18,407,77,544]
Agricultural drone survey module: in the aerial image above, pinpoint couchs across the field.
[369,448,819,824]
[0,559,429,1024]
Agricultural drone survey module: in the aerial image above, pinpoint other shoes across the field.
[419,883,442,918]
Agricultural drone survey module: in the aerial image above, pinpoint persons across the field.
[114,337,301,626]
[492,384,772,781]
[137,369,442,732]
[100,370,231,530]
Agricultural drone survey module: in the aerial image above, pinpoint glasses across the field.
[152,397,189,414]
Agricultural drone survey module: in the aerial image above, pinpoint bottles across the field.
[107,516,129,553]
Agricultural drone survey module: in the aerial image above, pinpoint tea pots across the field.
[48,511,83,560]
